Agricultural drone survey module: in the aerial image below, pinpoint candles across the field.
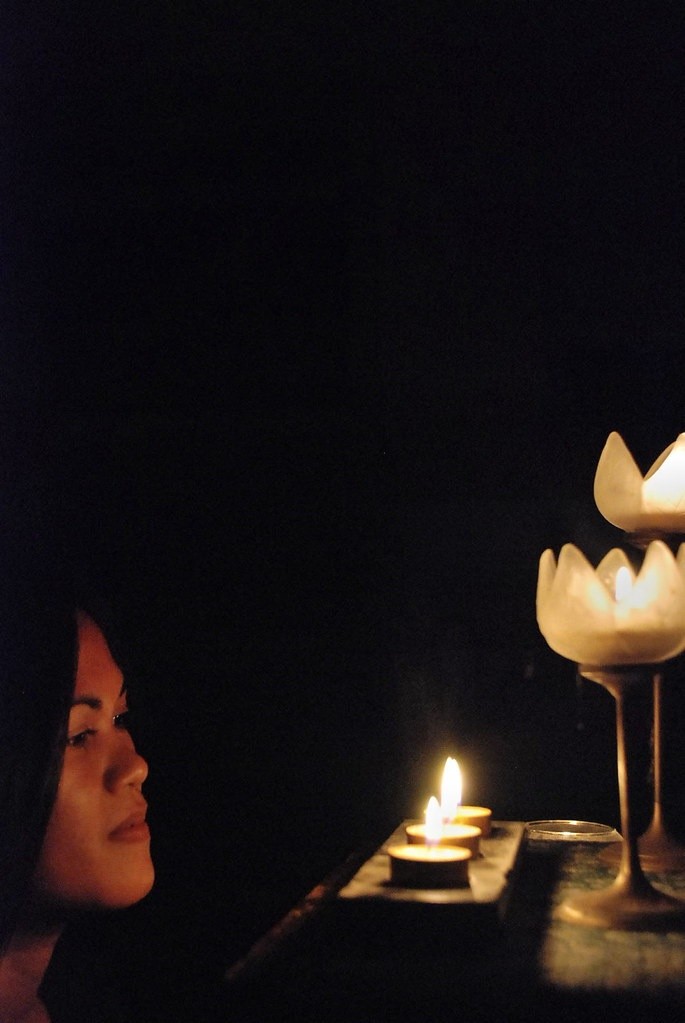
[423,806,491,836]
[388,845,472,888]
[537,539,685,668]
[406,823,480,858]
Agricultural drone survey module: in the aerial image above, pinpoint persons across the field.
[0,588,154,1023]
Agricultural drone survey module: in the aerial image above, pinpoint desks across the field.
[220,811,685,1023]
